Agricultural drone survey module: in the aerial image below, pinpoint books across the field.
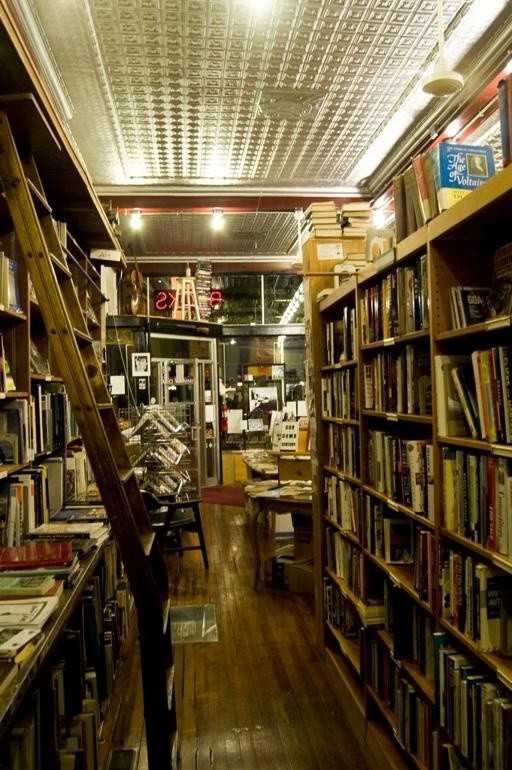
[362,345,431,415]
[322,475,361,537]
[321,369,355,420]
[323,307,356,365]
[0,443,112,696]
[440,447,511,557]
[326,527,364,600]
[0,383,81,465]
[323,575,433,769]
[433,631,512,770]
[359,254,428,345]
[393,142,496,241]
[304,201,371,239]
[435,346,512,445]
[326,423,359,478]
[437,550,502,654]
[0,540,136,770]
[365,494,434,607]
[366,429,433,522]
[448,286,493,329]
[0,251,51,391]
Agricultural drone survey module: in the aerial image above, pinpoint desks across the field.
[241,457,279,482]
[241,480,313,591]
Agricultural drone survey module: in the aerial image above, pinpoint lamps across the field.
[211,207,226,231]
[130,208,143,230]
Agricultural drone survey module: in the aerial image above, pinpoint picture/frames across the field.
[131,353,151,377]
[248,386,279,415]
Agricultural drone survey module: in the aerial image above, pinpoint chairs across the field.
[141,492,209,570]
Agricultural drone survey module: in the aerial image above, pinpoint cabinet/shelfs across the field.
[1,1,138,769]
[222,450,270,486]
[119,402,192,542]
[302,163,512,769]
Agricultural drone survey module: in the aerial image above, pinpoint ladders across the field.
[0,112,180,769]
